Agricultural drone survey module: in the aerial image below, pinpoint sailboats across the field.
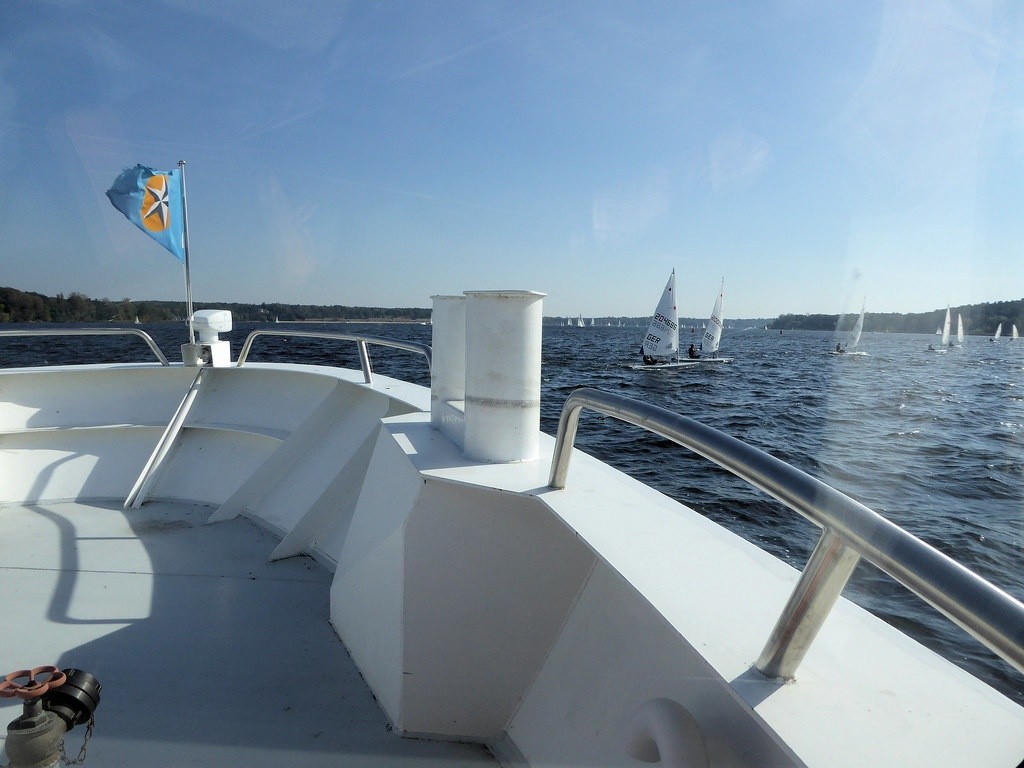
[274,315,280,324]
[560,313,612,328]
[632,268,700,371]
[1008,324,1020,342]
[949,313,966,349]
[134,316,143,325]
[671,276,735,364]
[830,308,868,356]
[989,322,1002,342]
[928,305,951,353]
[935,326,943,335]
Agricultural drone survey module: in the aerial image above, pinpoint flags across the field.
[104,163,186,262]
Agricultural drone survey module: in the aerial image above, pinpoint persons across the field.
[836,343,840,352]
[689,344,700,359]
[643,355,652,361]
